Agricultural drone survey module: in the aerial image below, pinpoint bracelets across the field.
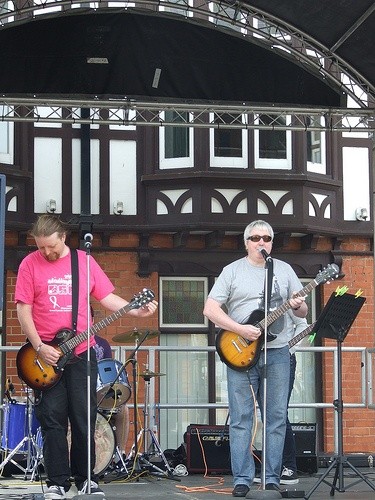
[35,342,44,354]
[293,306,301,311]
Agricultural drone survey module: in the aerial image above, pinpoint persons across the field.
[203,220,309,496]
[251,308,307,485]
[90,306,131,468]
[14,216,158,500]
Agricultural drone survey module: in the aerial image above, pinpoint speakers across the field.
[291,423,319,474]
[188,425,233,473]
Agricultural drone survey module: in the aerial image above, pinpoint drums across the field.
[36,410,117,480]
[95,358,132,410]
[1,396,41,455]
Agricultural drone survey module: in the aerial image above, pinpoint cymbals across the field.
[132,371,166,377]
[112,328,161,343]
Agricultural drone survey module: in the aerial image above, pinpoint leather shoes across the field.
[263,483,280,491]
[233,485,249,496]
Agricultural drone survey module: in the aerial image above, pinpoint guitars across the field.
[15,288,156,391]
[287,320,316,349]
[215,263,340,372]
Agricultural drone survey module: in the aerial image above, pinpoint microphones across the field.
[84,233,93,247]
[215,440,223,447]
[259,248,272,263]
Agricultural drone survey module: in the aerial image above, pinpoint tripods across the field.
[96,329,181,485]
[305,291,375,500]
[0,388,45,482]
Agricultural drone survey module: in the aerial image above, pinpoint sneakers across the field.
[78,479,105,495]
[44,485,65,500]
[253,474,263,483]
[280,467,299,484]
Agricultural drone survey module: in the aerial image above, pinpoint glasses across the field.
[247,235,272,243]
